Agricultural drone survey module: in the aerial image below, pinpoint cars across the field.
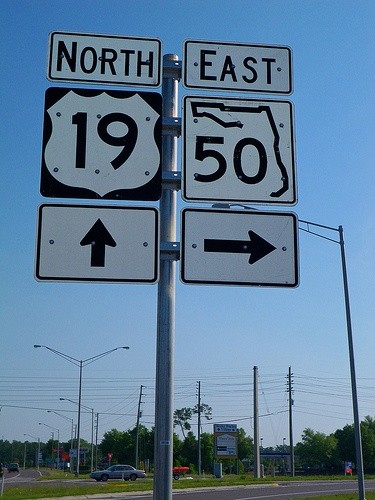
[172,466,190,480]
[0,463,10,477]
[89,464,147,482]
[7,463,20,472]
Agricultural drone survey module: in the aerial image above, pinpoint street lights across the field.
[59,397,93,473]
[47,411,74,472]
[38,422,60,469]
[33,344,132,477]
[283,438,286,450]
[260,438,264,447]
[24,433,40,472]
